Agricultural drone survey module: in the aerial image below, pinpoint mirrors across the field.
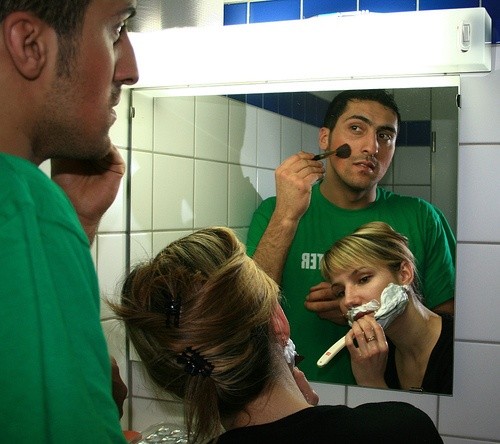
[127,84,459,397]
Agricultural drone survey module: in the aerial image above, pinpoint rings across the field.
[367,335,376,342]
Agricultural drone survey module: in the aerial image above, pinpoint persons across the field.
[106,227,445,444]
[0,0,139,443]
[320,221,453,394]
[245,90,456,384]
